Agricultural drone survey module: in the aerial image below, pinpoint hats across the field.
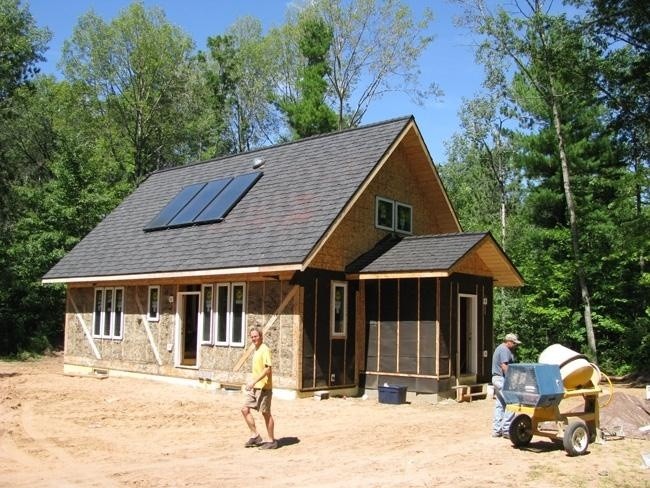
[505,333,522,344]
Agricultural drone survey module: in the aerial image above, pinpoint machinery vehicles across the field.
[499,343,605,459]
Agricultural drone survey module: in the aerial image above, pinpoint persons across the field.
[491,333,522,439]
[241,327,279,450]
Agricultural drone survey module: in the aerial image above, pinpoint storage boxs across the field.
[377,385,407,407]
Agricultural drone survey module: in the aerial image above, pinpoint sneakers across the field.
[245,434,262,447]
[259,440,278,450]
